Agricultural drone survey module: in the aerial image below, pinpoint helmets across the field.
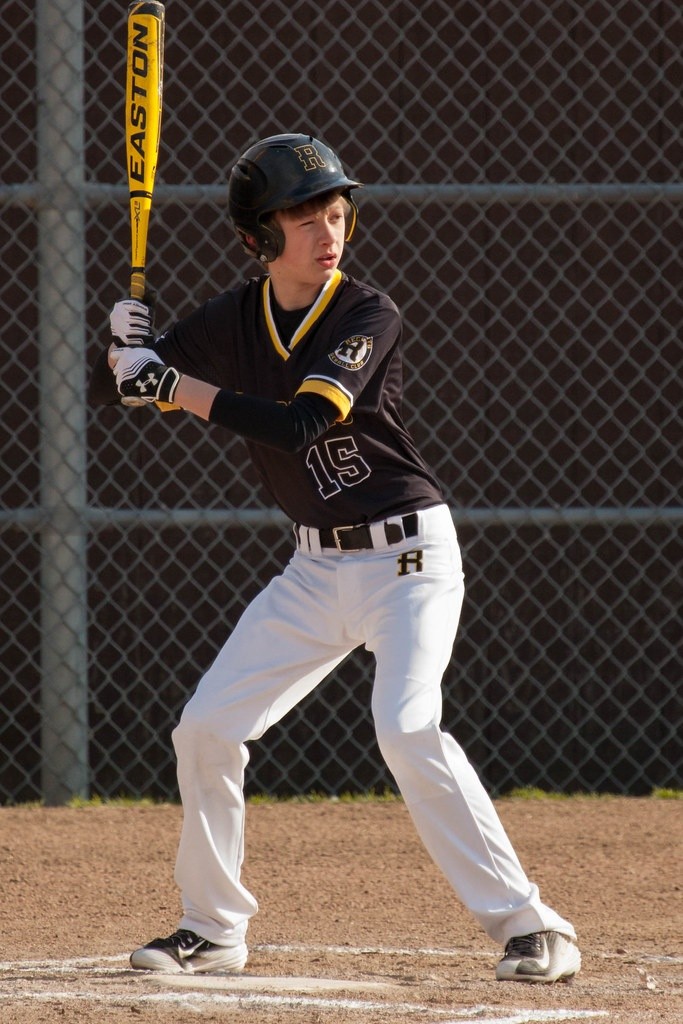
[227,133,363,261]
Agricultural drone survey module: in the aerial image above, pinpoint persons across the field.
[108,131,584,985]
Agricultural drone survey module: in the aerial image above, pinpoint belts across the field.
[296,514,418,553]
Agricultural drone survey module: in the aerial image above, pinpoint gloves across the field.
[109,348,183,403]
[109,298,153,346]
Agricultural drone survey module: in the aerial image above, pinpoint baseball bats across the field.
[119,18,168,412]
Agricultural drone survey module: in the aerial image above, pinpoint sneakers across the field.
[129,930,249,973]
[496,931,580,984]
